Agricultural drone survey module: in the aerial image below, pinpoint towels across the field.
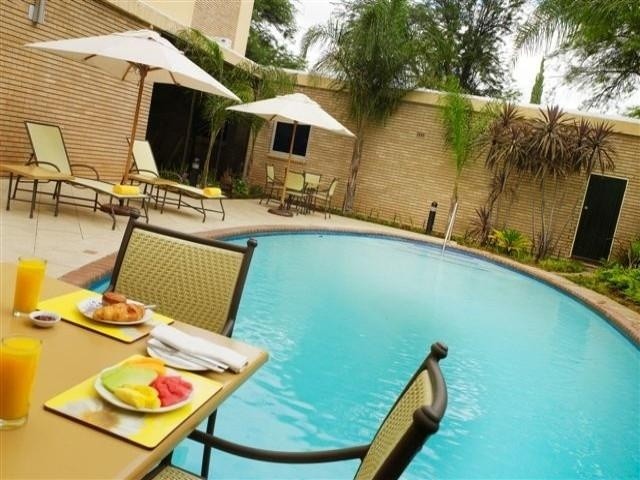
[204,187,222,196]
[113,184,139,195]
[149,322,249,373]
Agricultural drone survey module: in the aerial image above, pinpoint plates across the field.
[77,296,223,413]
[29,310,61,327]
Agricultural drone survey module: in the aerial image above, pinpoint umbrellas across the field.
[24,28,241,214]
[225,93,356,216]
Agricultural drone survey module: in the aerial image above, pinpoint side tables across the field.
[0,164,75,219]
[127,174,177,214]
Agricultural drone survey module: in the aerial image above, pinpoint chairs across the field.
[13,122,150,229]
[142,340,448,480]
[106,207,258,480]
[120,136,228,223]
[259,163,340,219]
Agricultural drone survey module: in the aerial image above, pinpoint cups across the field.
[12,255,47,318]
[0,333,44,430]
